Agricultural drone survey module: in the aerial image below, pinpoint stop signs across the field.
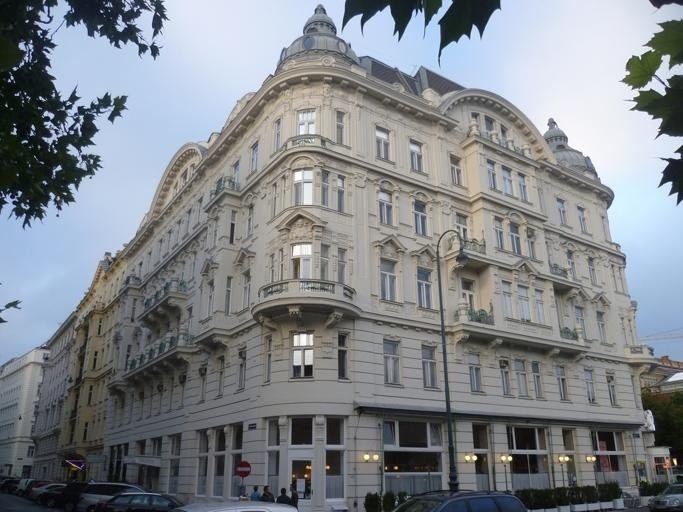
[236,461,250,476]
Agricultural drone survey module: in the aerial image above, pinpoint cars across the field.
[41,485,67,509]
[88,493,184,512]
[168,501,298,512]
[0,476,53,499]
[30,482,67,507]
[647,483,683,511]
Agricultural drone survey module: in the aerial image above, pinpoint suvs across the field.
[391,489,526,512]
[63,481,102,511]
[75,479,151,511]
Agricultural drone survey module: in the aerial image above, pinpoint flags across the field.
[66,459,84,470]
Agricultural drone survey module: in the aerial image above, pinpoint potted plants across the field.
[514,478,652,512]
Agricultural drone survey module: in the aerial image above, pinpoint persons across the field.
[289,484,298,508]
[260,485,275,503]
[250,485,262,500]
[276,488,291,505]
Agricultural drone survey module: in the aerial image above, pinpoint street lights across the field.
[557,455,569,487]
[501,454,512,490]
[437,229,468,488]
[584,456,600,489]
[666,455,677,483]
